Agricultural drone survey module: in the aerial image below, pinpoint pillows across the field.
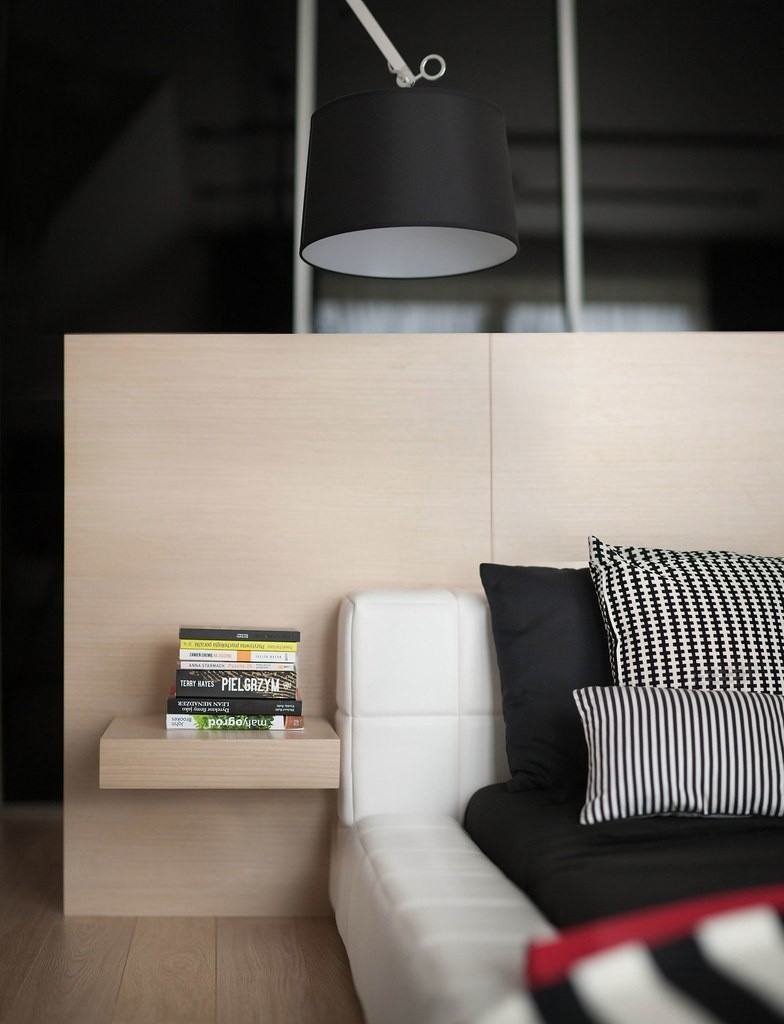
[572,687,784,824]
[479,562,613,794]
[588,534,784,694]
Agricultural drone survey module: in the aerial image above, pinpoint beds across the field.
[321,585,784,1024]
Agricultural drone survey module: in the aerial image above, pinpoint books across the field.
[165,626,307,731]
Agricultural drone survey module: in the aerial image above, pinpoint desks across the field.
[97,716,342,791]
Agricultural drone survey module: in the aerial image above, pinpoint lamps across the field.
[300,0,522,278]
